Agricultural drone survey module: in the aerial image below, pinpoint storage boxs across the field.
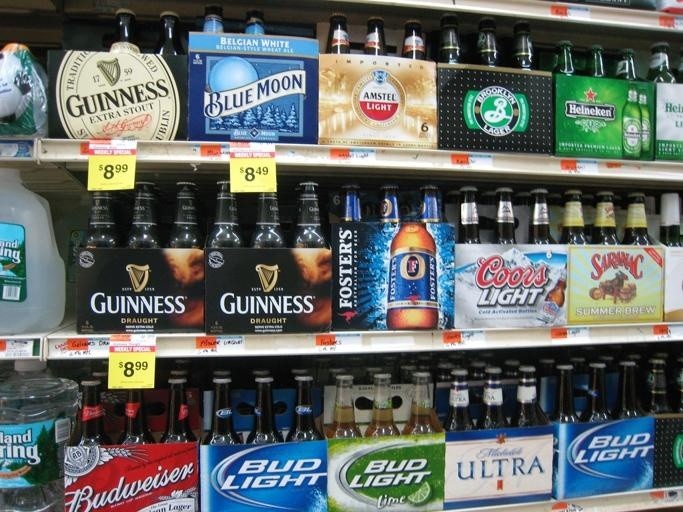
[57,32,683,512]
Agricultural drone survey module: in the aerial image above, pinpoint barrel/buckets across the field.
[0,164,67,335]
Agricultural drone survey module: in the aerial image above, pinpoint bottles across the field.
[77,347,682,447]
[534,257,567,322]
[451,182,683,244]
[384,222,442,330]
[335,181,448,222]
[109,4,267,56]
[83,175,327,248]
[325,10,683,82]
[621,81,651,158]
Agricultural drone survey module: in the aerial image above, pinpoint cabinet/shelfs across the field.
[0,131,87,359]
[37,1,683,509]
